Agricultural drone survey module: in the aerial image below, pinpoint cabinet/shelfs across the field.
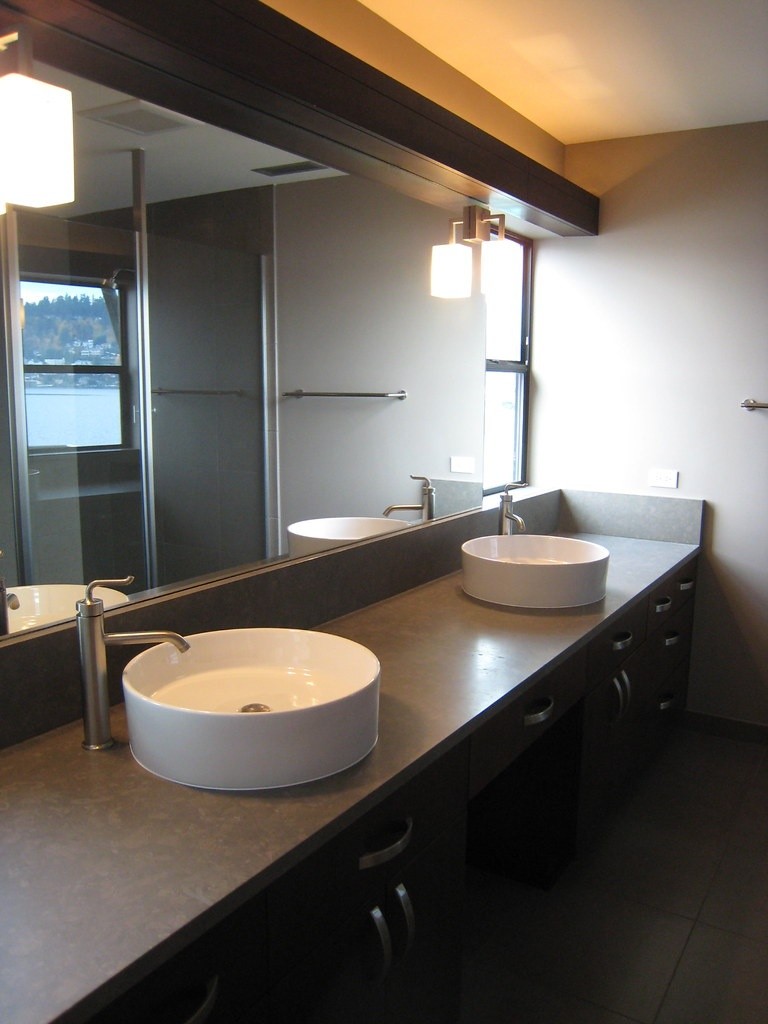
[85,560,699,1024]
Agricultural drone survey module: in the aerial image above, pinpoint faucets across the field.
[498,482,528,535]
[383,473,437,523]
[0,546,20,635]
[73,573,191,751]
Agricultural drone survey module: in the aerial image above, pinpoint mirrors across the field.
[0,8,489,646]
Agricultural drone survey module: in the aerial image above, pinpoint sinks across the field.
[459,533,610,609]
[286,516,414,558]
[122,627,380,791]
[2,582,130,640]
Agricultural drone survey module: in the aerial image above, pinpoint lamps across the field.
[431,206,476,300]
[0,20,75,209]
[469,204,522,299]
